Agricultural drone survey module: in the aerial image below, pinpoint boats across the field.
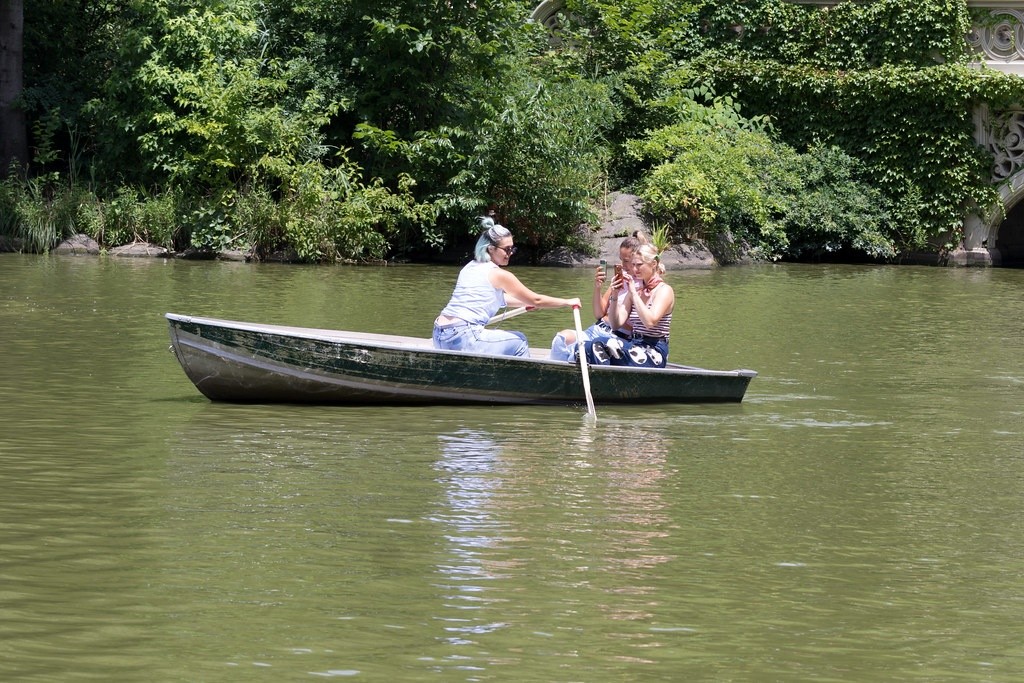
[162,313,759,408]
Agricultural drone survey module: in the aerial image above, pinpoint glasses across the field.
[493,245,517,255]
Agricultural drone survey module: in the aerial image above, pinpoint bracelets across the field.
[609,296,618,301]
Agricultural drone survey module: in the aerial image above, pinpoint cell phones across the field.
[599,259,608,282]
[614,264,624,287]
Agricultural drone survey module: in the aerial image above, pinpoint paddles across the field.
[572,303,597,422]
[483,306,535,327]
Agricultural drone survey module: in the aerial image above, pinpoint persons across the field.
[551,230,675,368]
[432,216,582,358]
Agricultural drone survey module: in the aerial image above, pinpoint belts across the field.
[633,333,665,342]
[595,319,631,342]
